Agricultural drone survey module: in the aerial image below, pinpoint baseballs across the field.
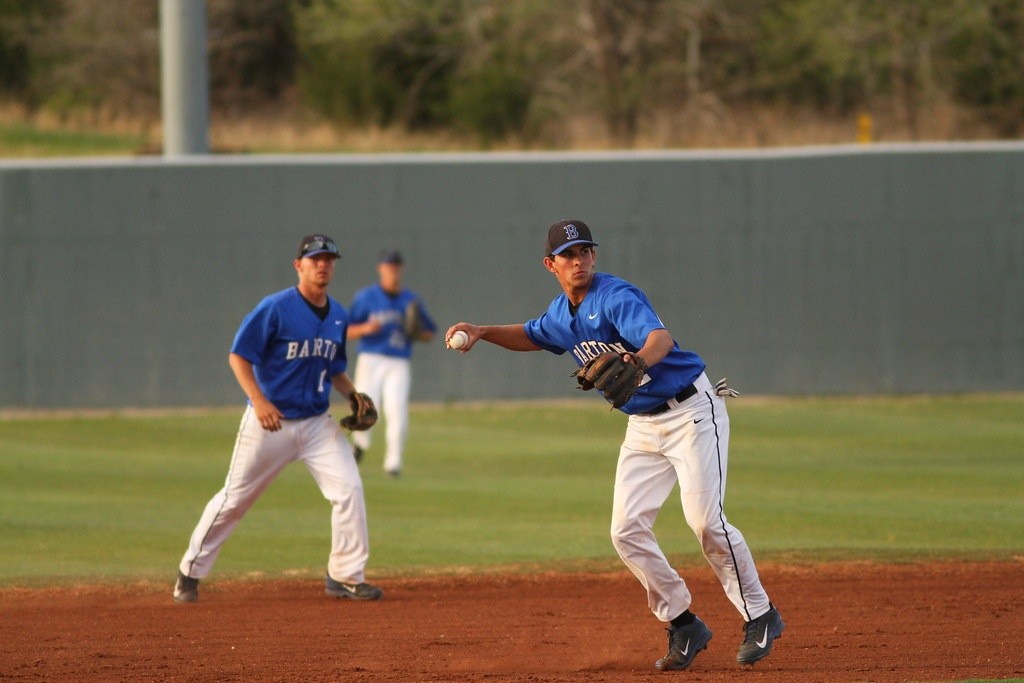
[448,330,469,350]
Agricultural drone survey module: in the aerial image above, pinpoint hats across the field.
[376,249,404,265]
[546,219,599,257]
[295,233,341,261]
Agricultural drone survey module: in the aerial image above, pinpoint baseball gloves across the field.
[339,391,378,432]
[577,351,649,410]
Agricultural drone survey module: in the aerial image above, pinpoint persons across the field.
[445,220,785,670]
[347,250,438,476]
[173,235,382,603]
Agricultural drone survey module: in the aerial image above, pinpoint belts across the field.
[640,383,697,415]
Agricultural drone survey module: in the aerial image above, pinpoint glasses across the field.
[310,253,333,262]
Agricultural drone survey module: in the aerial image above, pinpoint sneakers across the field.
[324,572,383,602]
[173,571,198,603]
[735,602,785,665]
[654,613,714,672]
